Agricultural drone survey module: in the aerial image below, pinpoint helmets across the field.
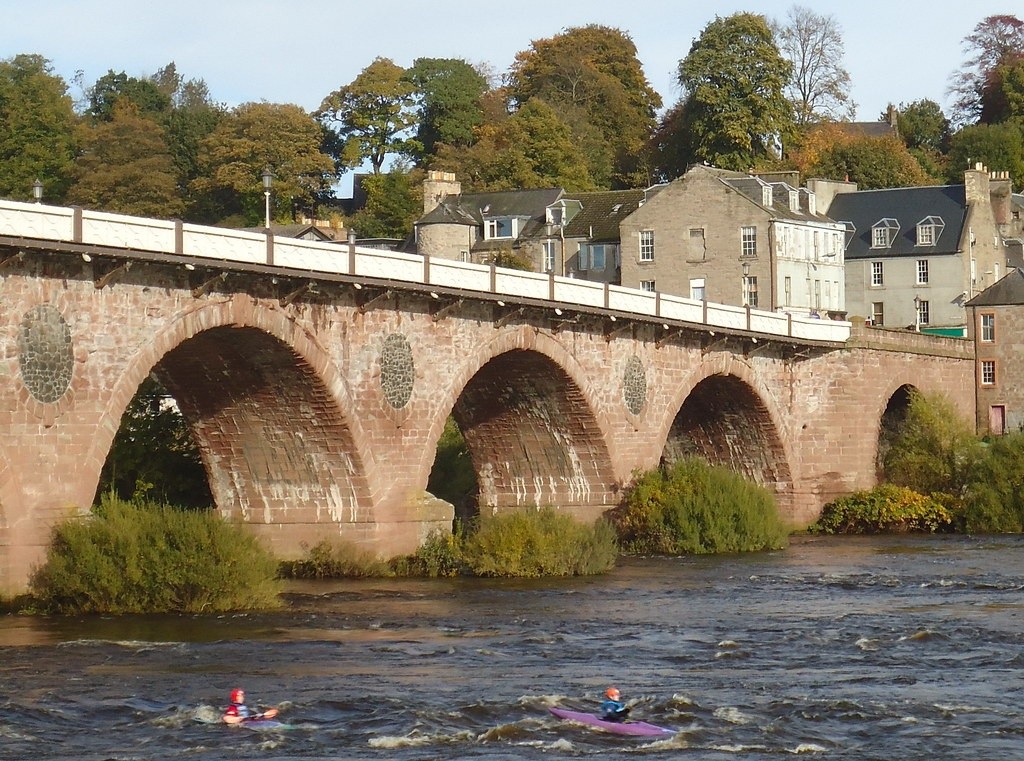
[230,687,247,702]
[604,687,620,699]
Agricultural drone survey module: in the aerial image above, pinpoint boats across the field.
[191,715,297,731]
[545,705,678,737]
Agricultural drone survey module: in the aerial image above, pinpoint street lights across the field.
[913,295,922,332]
[260,171,272,228]
[742,261,751,305]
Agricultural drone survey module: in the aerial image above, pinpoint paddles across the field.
[222,709,278,724]
[630,699,644,709]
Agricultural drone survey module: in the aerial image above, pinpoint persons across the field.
[601,688,644,723]
[223,689,265,723]
